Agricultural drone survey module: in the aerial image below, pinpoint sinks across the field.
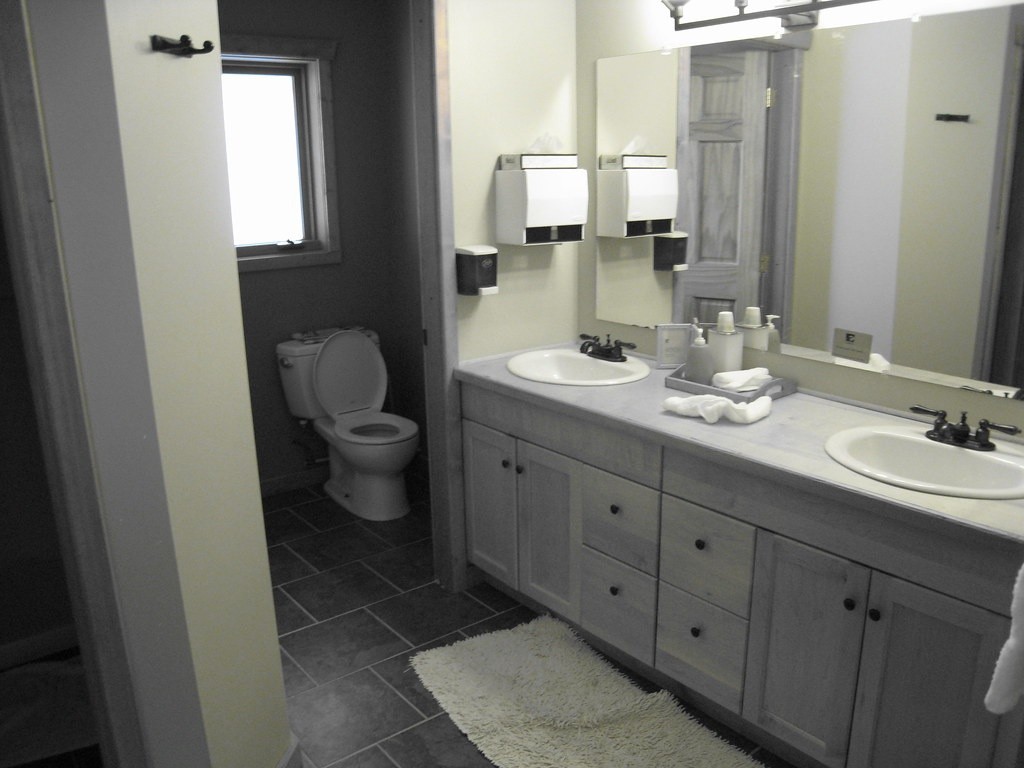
[506,347,650,387]
[976,388,1022,399]
[825,424,1024,500]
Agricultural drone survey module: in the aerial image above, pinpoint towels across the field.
[834,352,892,374]
[983,561,1023,713]
[711,366,772,392]
[661,393,773,425]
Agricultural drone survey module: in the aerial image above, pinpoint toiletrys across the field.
[764,315,781,354]
[686,324,714,385]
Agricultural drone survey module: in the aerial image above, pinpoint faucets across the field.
[579,341,609,356]
[1010,388,1024,398]
[939,422,968,446]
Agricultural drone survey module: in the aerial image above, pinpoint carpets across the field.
[0,656,99,768]
[409,613,766,768]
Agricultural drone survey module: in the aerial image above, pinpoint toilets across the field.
[275,324,421,521]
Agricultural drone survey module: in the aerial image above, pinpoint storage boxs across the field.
[497,167,588,246]
[598,169,678,238]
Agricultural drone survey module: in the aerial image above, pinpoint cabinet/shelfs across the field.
[462,385,1024,768]
